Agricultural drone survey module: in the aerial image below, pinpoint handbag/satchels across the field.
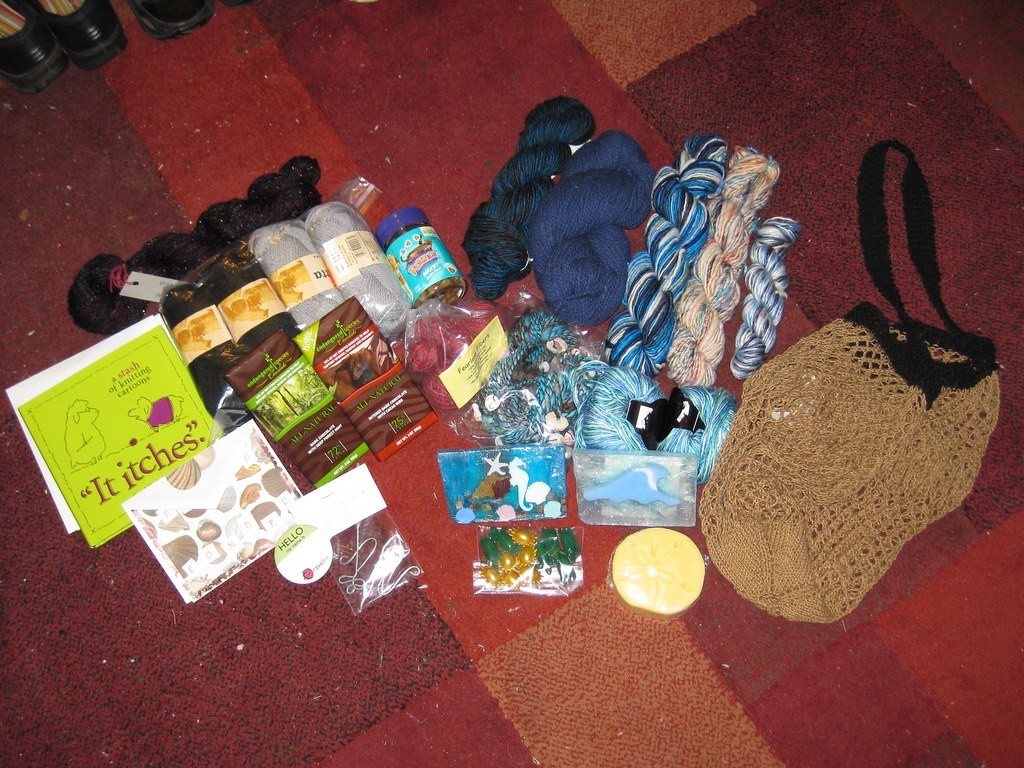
[699,139,1000,624]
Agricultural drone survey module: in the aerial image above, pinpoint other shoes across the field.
[33,0,127,71]
[0,0,70,96]
[127,0,256,41]
